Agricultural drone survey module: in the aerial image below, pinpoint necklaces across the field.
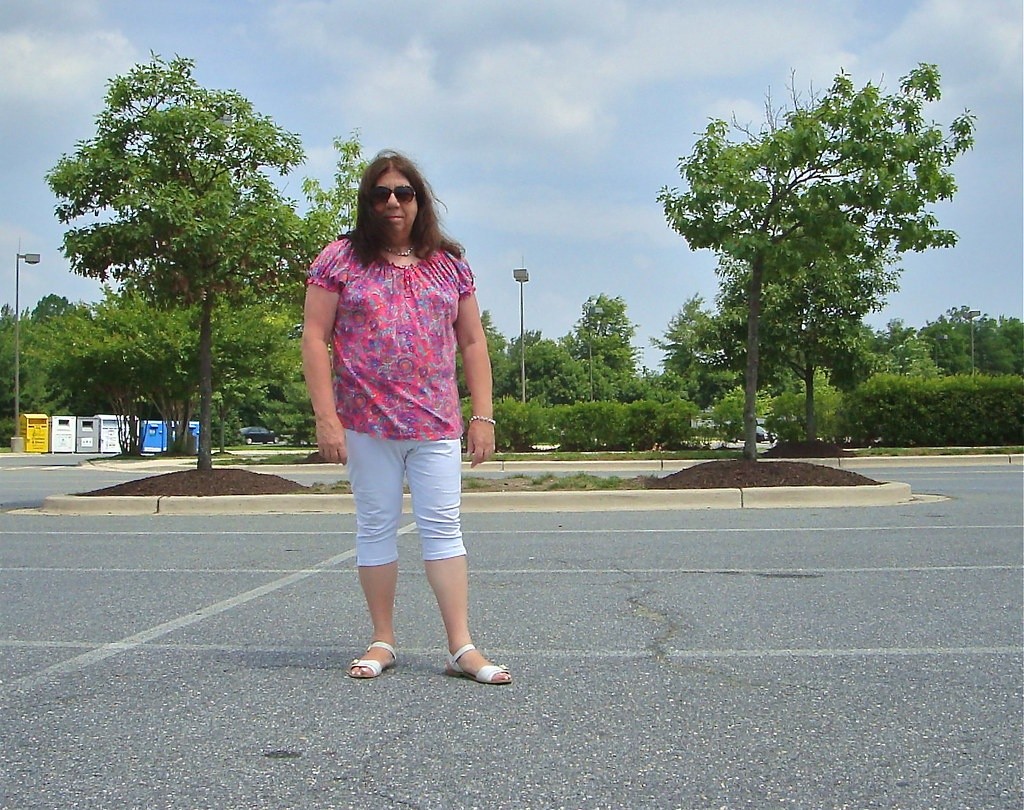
[385,245,415,256]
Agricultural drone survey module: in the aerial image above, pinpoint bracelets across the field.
[469,416,496,426]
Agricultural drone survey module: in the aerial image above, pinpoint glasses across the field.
[371,186,417,204]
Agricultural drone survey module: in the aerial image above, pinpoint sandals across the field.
[348,642,397,678]
[446,644,512,684]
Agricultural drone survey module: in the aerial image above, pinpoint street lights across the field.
[963,311,980,374]
[15,252,39,439]
[588,297,603,400]
[513,268,528,401]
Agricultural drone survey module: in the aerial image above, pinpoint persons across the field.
[299,154,514,685]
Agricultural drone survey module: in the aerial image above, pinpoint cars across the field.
[239,427,281,445]
[737,426,777,444]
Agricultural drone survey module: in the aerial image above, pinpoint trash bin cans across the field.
[95,415,139,455]
[76,416,100,453]
[49,415,76,454]
[19,413,49,455]
[140,420,200,454]
